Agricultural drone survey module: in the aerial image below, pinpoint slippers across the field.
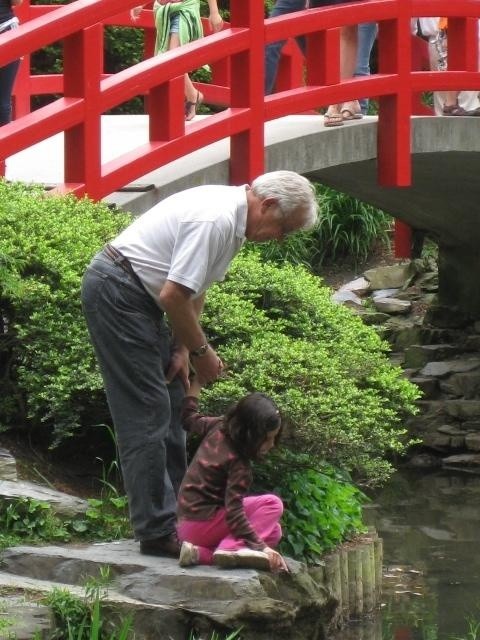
[324,108,365,127]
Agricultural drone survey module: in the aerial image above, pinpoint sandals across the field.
[445,97,467,116]
[186,89,203,120]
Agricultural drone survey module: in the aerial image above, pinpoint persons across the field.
[176,361,290,572]
[131,0,226,121]
[0,0,23,128]
[262,1,480,126]
[83,170,323,554]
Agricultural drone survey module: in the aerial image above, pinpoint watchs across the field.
[191,342,211,357]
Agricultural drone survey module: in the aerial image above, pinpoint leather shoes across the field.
[140,532,181,558]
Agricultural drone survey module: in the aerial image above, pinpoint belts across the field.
[105,242,133,274]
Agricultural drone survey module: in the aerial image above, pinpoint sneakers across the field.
[178,541,200,566]
[214,548,270,570]
